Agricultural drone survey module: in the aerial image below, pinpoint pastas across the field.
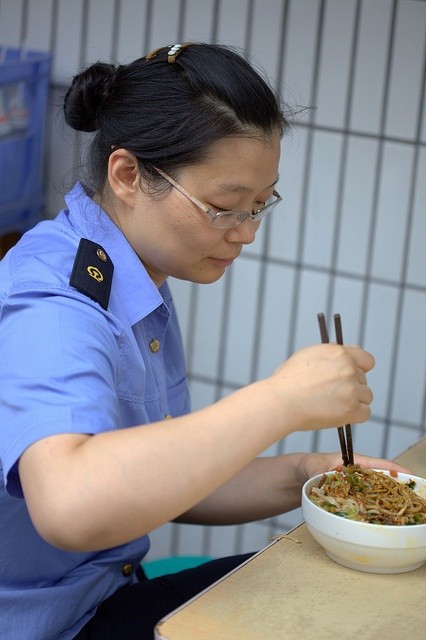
[308,464,426,525]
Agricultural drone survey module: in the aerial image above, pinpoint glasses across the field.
[109,145,284,231]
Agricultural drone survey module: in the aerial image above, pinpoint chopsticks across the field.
[316,312,363,467]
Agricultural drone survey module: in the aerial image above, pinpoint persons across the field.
[0,39,419,634]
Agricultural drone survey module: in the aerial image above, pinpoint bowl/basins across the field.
[299,462,426,575]
